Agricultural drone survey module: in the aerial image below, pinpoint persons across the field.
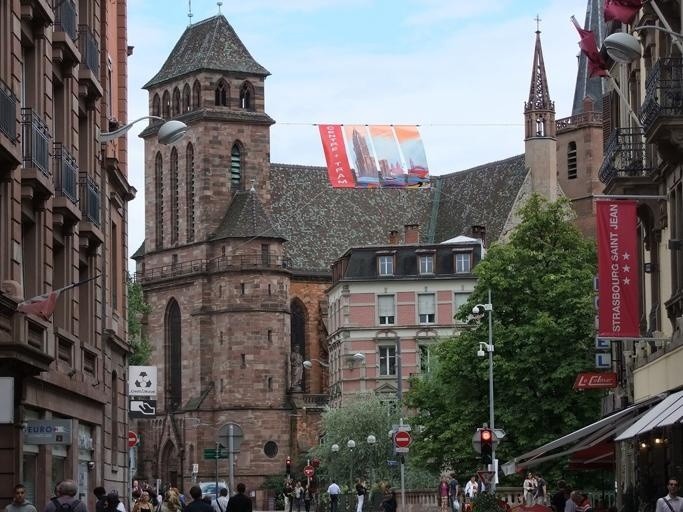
[290,344,303,387]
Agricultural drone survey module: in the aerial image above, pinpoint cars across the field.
[201,484,222,494]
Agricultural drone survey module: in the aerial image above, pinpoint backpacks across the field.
[51,498,81,512]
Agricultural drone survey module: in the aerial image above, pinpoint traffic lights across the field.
[284,459,290,475]
[479,430,493,465]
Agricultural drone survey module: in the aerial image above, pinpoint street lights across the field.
[331,443,339,486]
[471,289,498,494]
[346,439,355,491]
[366,435,376,490]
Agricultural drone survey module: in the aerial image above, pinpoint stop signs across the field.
[393,431,411,448]
[128,431,136,448]
[303,466,315,477]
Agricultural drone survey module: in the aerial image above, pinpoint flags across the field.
[604,0,642,24]
[575,26,610,79]
[16,289,60,322]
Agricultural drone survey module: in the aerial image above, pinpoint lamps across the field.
[354,351,365,365]
[302,359,329,370]
[98,111,188,148]
[603,25,683,64]
